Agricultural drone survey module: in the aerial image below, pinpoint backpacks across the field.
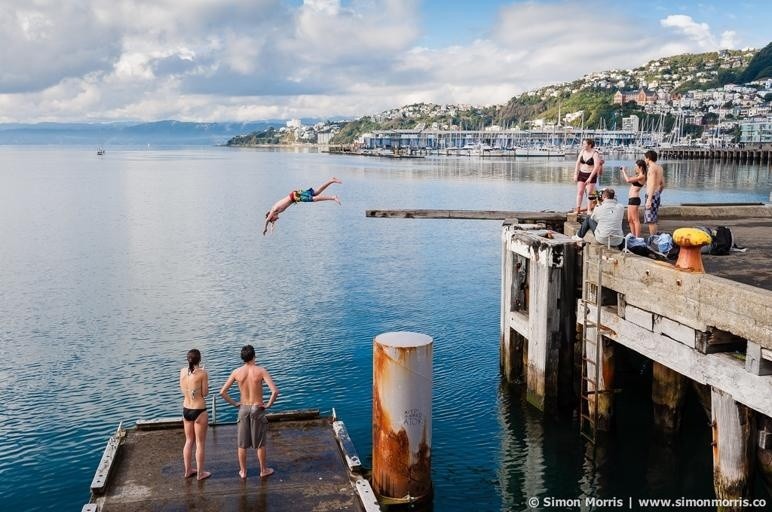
[708,226,732,256]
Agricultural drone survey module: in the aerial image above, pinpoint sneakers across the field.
[572,234,583,241]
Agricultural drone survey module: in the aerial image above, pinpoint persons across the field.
[573,138,599,215]
[645,151,664,236]
[220,345,280,479]
[594,151,605,188]
[262,176,343,235]
[620,160,648,237]
[571,190,624,249]
[178,349,211,481]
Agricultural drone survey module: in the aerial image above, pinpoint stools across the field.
[674,228,714,273]
